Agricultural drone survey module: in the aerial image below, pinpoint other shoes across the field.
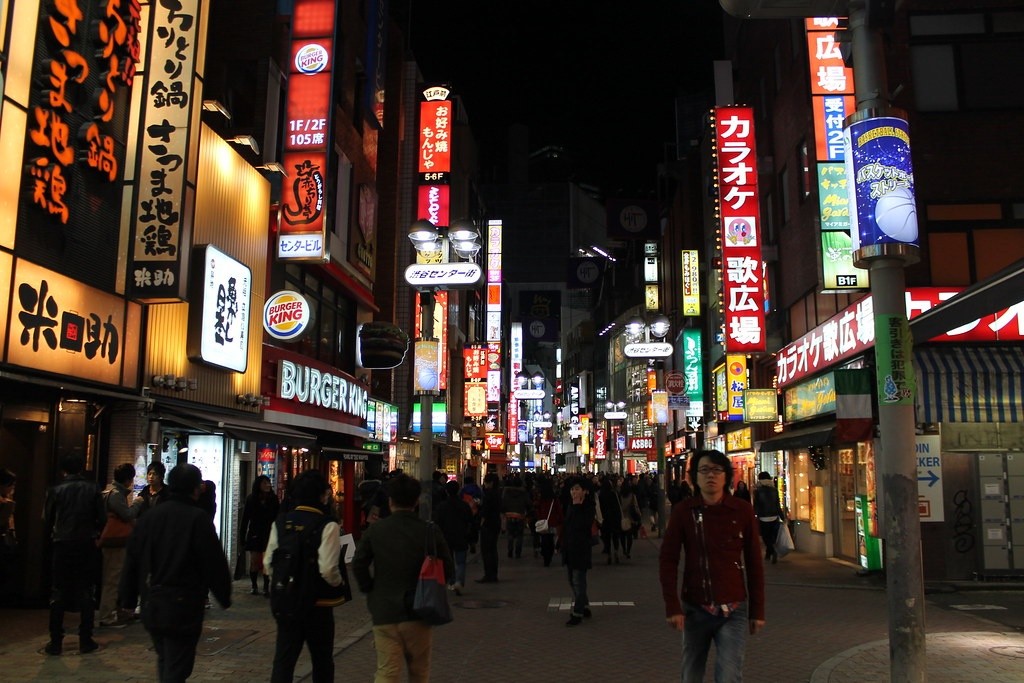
[45,641,63,655]
[566,616,583,626]
[447,583,463,596]
[79,639,99,653]
[507,546,635,567]
[771,553,778,564]
[569,609,593,620]
[99,611,127,629]
[474,576,499,584]
[765,553,771,561]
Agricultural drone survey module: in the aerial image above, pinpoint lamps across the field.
[254,161,287,177]
[175,436,188,453]
[241,440,250,454]
[360,373,369,382]
[599,322,622,336]
[202,100,231,119]
[225,135,259,155]
[238,394,271,408]
[154,374,197,391]
[86,400,104,418]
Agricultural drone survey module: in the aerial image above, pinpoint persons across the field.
[39,448,788,683]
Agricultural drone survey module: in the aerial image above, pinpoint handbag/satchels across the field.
[535,519,549,533]
[411,521,454,627]
[621,517,632,531]
[95,488,135,549]
[774,521,795,557]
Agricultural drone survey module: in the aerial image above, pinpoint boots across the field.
[262,573,270,595]
[250,569,258,594]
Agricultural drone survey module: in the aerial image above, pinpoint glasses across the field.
[696,465,725,475]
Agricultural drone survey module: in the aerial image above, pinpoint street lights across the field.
[624,322,669,541]
[403,227,485,523]
[603,401,627,460]
